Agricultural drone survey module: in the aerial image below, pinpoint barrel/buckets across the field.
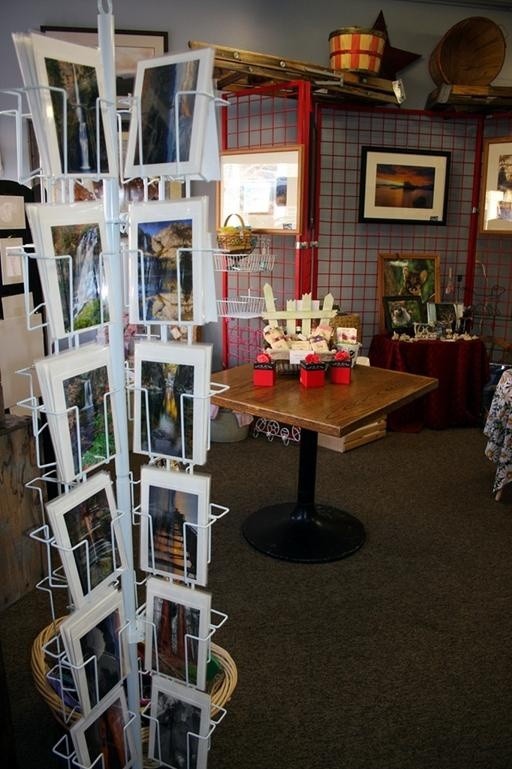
[429,15,506,89]
[329,25,386,73]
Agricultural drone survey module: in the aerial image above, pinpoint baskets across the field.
[217,213,257,253]
[213,253,275,273]
[29,615,238,769]
[216,296,277,316]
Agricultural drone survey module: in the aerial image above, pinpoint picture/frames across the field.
[39,23,168,133]
[358,145,452,227]
[213,142,305,237]
[477,135,512,236]
[376,249,456,335]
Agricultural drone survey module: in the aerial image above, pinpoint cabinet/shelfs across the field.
[1,1,231,768]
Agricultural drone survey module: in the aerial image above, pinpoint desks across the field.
[209,359,440,563]
[366,334,491,434]
